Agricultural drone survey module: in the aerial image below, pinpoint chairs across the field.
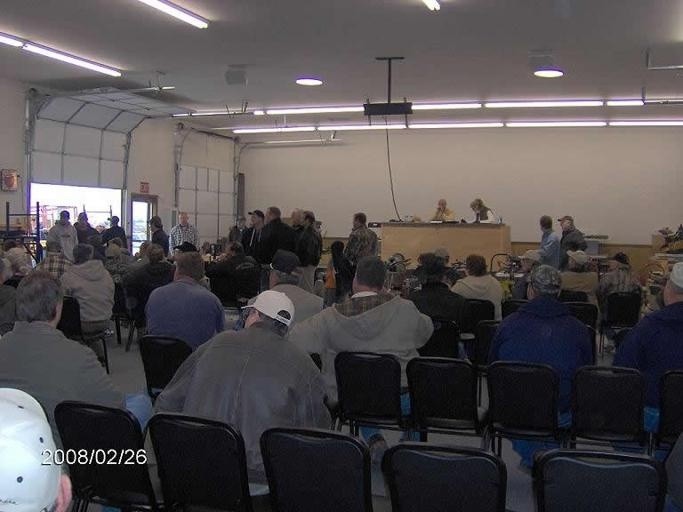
[646,368,683,460]
[566,363,648,450]
[500,295,531,320]
[471,319,496,370]
[53,398,165,512]
[556,300,600,331]
[330,351,410,437]
[406,353,483,442]
[556,287,591,303]
[141,412,253,511]
[112,311,129,345]
[604,290,642,332]
[137,335,193,399]
[484,357,568,458]
[461,299,496,327]
[56,295,110,374]
[381,443,508,512]
[416,313,460,357]
[125,319,137,352]
[259,426,373,512]
[532,449,587,511]
[4,276,26,290]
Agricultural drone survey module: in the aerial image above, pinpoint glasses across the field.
[236,217,246,222]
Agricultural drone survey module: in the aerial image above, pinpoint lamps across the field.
[0,31,124,88]
[170,94,682,121]
[230,119,683,137]
[139,0,211,35]
[294,72,325,86]
[531,62,565,81]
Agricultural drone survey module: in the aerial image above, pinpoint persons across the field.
[611,260,683,460]
[451,254,503,340]
[48,210,77,263]
[0,236,32,268]
[241,210,265,257]
[535,214,560,267]
[102,215,128,249]
[408,256,468,361]
[157,288,332,501]
[487,263,593,470]
[229,215,248,242]
[292,254,434,475]
[297,211,323,293]
[1,385,73,512]
[510,250,541,301]
[104,244,129,284]
[148,215,169,255]
[109,238,130,264]
[213,241,259,307]
[87,234,104,264]
[145,251,225,357]
[234,250,324,337]
[59,243,116,363]
[168,211,198,258]
[0,270,151,466]
[432,199,455,223]
[258,206,296,289]
[597,251,641,352]
[559,250,598,304]
[173,242,212,291]
[557,215,587,270]
[470,198,499,224]
[0,259,16,335]
[122,244,173,314]
[74,211,98,243]
[435,247,459,281]
[343,213,379,267]
[30,241,74,281]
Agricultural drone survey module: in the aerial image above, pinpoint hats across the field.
[566,249,587,264]
[105,244,120,257]
[557,215,573,224]
[147,215,162,226]
[525,265,565,287]
[670,262,683,287]
[608,252,629,265]
[435,249,450,260]
[107,216,118,222]
[174,242,197,253]
[248,210,264,220]
[518,249,542,263]
[240,290,294,327]
[0,388,62,512]
